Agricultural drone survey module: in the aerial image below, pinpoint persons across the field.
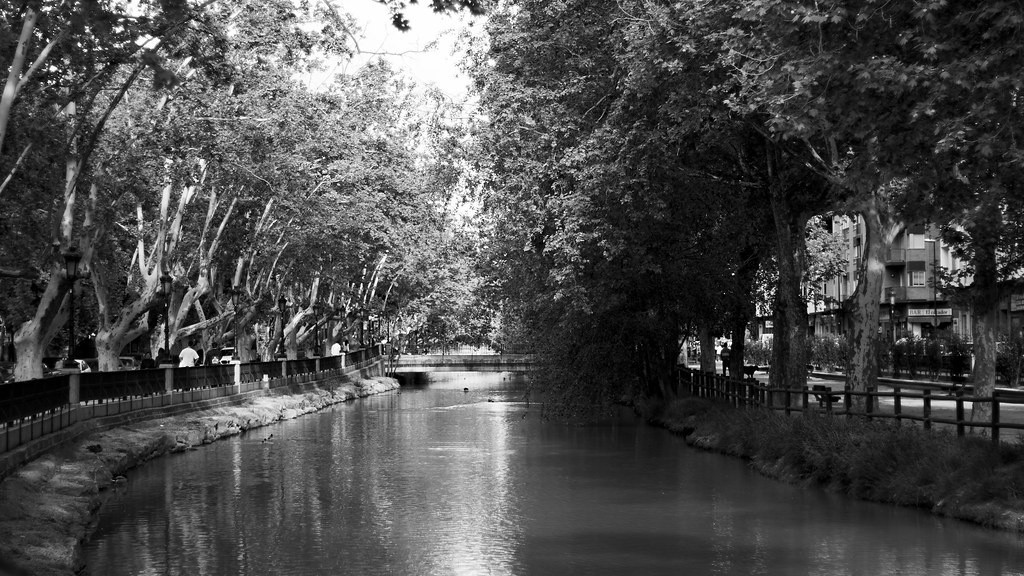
[721,343,731,376]
[178,340,199,367]
[368,336,376,347]
[331,341,341,355]
[153,347,168,368]
[140,352,157,370]
[205,342,223,387]
[342,339,350,353]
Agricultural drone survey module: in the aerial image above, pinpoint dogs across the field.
[743,365,760,378]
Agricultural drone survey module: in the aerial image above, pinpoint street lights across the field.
[925,238,937,340]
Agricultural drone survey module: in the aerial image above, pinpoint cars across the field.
[119,356,140,371]
[0,361,60,385]
[218,347,236,364]
[53,358,92,375]
